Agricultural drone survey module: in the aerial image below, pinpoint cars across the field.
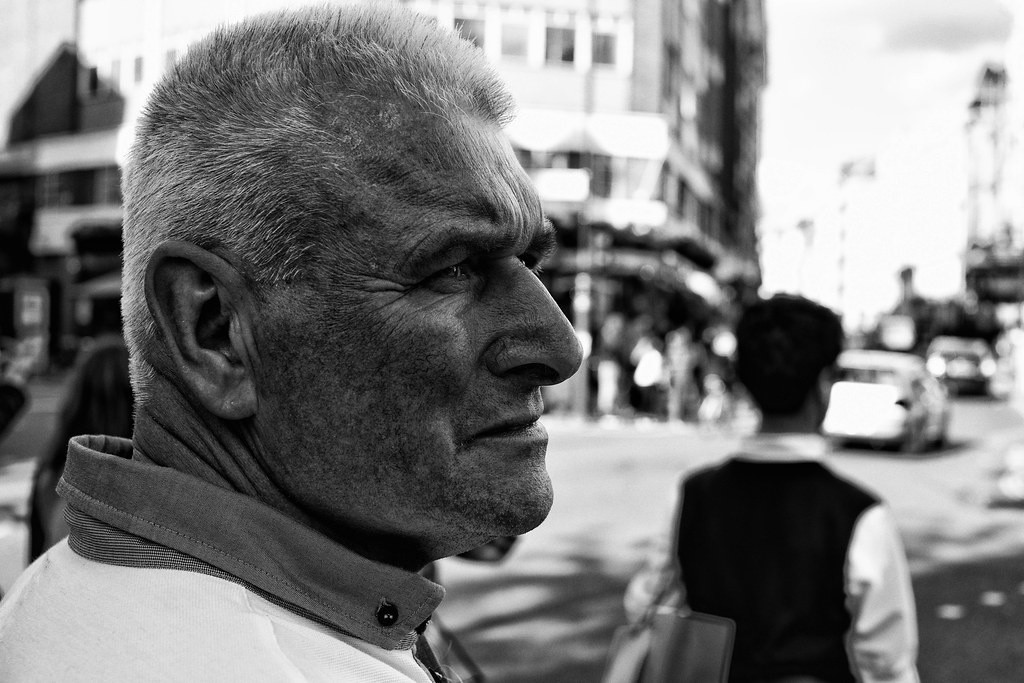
[927,335,999,398]
[826,348,955,455]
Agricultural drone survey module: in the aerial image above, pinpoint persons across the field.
[623,290,920,683]
[0,0,581,682]
[26,339,138,558]
